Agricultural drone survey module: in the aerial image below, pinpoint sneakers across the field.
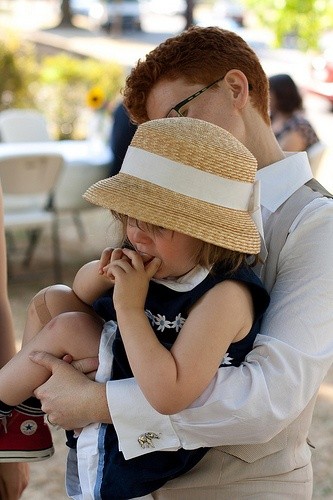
[0,410,55,463]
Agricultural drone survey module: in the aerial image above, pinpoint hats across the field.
[82,118,261,254]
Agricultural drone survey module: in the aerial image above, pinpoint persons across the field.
[0,117,271,500]
[0,26,332,500]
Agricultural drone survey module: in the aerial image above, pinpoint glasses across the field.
[164,76,224,117]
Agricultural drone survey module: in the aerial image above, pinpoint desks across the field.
[0,142,108,214]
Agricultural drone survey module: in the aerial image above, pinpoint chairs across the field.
[0,154,64,289]
[0,108,88,244]
